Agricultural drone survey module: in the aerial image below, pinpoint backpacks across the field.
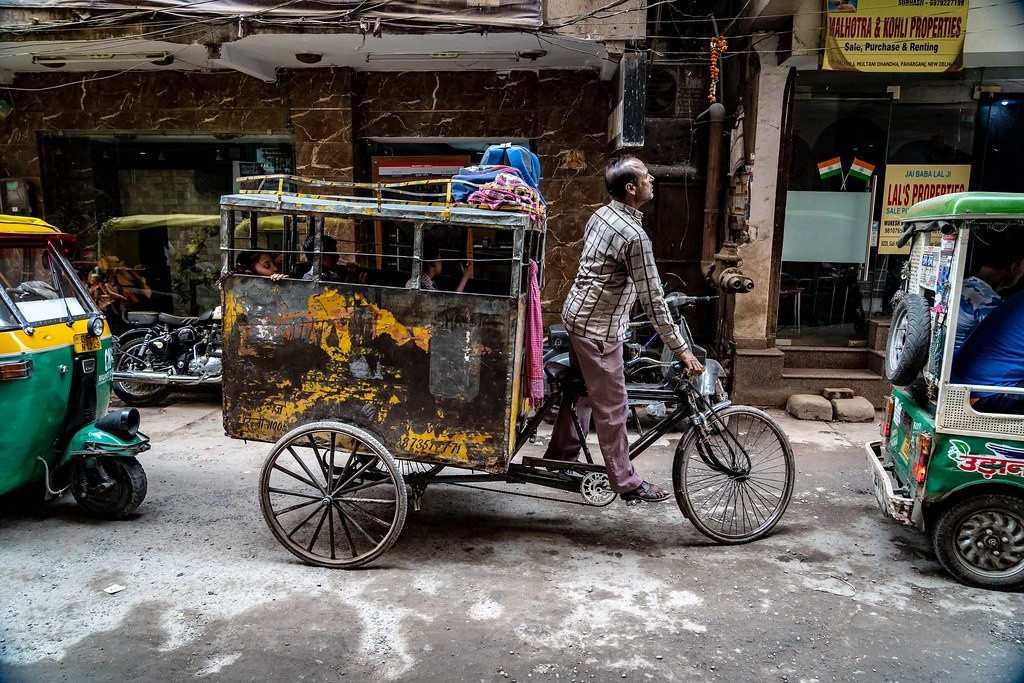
[478,144,548,207]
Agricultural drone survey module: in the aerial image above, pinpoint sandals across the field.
[546,460,588,475]
[620,481,671,502]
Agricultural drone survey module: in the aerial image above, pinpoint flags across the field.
[848,159,876,182]
[817,156,843,180]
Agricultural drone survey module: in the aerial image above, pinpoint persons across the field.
[950,224,1024,387]
[404,238,472,292]
[542,157,706,503]
[236,247,290,281]
[303,234,359,283]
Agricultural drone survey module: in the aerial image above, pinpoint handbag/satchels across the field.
[452,165,547,229]
[660,315,694,384]
